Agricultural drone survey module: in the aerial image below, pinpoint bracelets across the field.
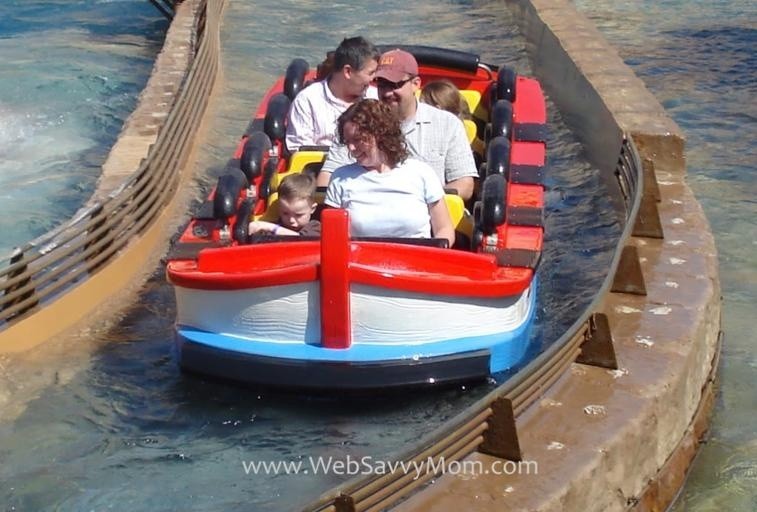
[272,226,282,236]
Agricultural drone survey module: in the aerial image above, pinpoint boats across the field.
[164,43,549,395]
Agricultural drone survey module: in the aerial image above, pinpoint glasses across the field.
[375,77,414,89]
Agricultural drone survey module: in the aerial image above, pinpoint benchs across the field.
[253,89,488,244]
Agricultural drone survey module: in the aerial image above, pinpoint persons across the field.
[417,81,488,160]
[247,171,323,238]
[280,35,382,154]
[318,96,456,249]
[368,46,479,200]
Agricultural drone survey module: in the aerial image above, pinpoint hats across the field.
[372,48,419,84]
